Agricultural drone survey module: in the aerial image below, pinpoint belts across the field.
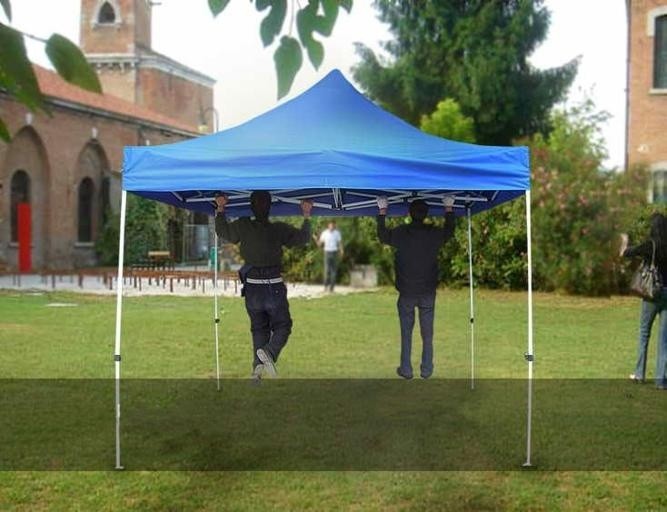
[246,277,283,284]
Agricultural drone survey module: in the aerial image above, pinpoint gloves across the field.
[376,195,389,209]
[442,195,456,207]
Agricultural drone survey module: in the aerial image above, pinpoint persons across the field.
[619,211,667,391]
[376,194,455,381]
[313,220,344,293]
[216,190,312,385]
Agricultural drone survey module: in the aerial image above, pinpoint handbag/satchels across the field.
[630,259,664,302]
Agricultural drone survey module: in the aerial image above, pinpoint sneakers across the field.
[252,364,264,377]
[629,374,643,384]
[256,349,278,378]
[397,367,412,379]
